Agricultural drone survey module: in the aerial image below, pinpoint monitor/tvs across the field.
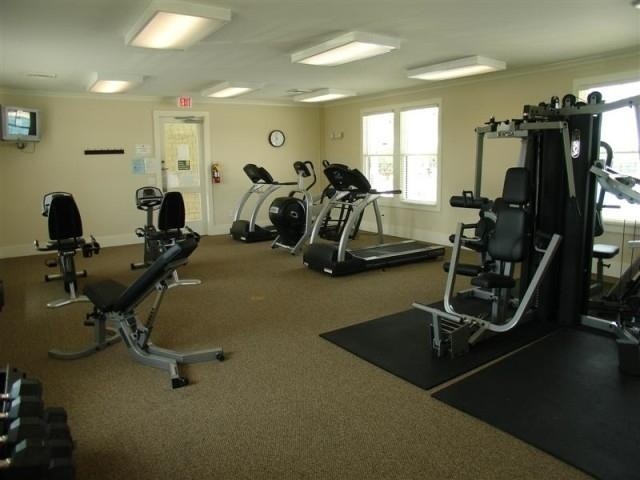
[0,106,42,140]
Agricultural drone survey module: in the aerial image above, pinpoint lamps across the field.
[123,1,233,55]
[202,81,264,100]
[86,71,132,96]
[289,29,508,104]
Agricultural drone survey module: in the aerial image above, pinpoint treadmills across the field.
[230,164,316,243]
[302,159,446,278]
